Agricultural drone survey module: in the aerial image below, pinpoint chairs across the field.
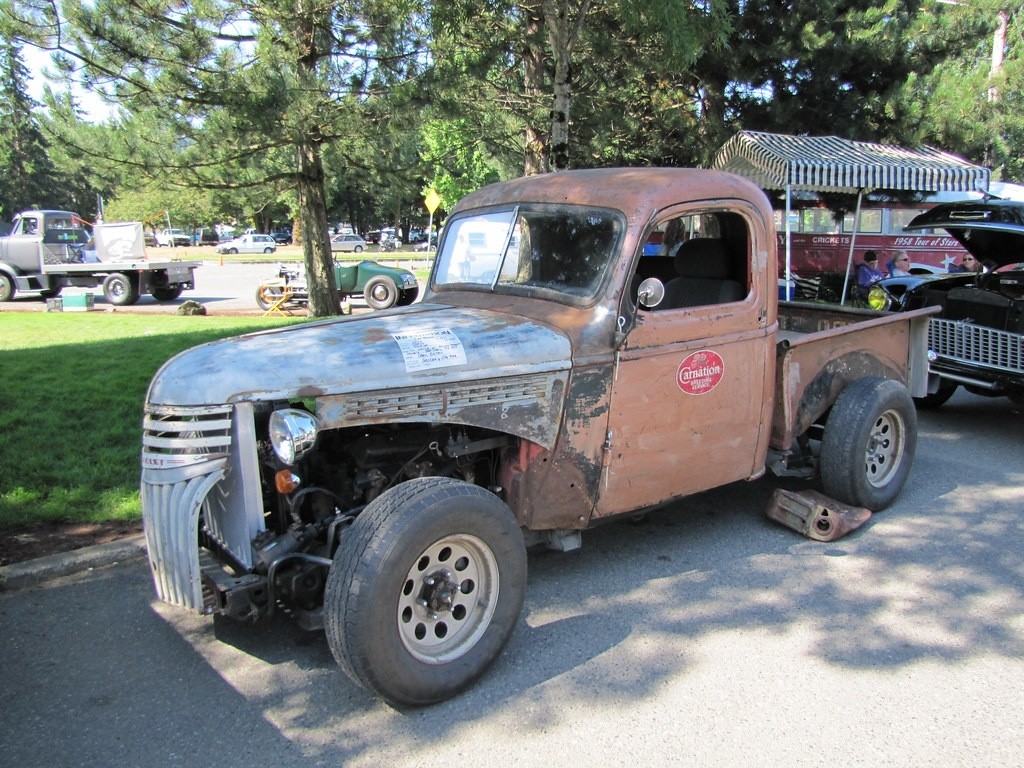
[255,272,294,319]
[647,237,747,311]
[886,259,896,277]
[852,259,881,309]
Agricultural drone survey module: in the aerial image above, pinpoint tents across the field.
[699,130,991,311]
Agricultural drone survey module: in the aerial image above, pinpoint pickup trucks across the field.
[139,163,945,709]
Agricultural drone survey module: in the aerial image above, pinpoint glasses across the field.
[899,257,910,261]
[962,258,973,261]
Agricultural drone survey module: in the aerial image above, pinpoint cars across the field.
[864,196,1023,421]
[328,234,369,254]
[216,233,277,256]
[268,232,292,247]
[258,260,422,315]
[364,226,437,245]
[142,232,157,249]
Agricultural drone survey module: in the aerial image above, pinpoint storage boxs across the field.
[61,292,94,312]
[778,279,795,300]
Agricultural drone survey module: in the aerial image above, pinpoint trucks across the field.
[0,209,206,304]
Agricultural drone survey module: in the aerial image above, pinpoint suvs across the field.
[156,228,192,247]
[189,226,219,248]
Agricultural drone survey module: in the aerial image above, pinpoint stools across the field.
[68,243,86,264]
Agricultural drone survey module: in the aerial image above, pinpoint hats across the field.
[864,250,877,260]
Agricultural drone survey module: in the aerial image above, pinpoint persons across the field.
[657,218,684,257]
[856,250,886,287]
[955,252,981,273]
[457,236,471,280]
[885,249,911,278]
